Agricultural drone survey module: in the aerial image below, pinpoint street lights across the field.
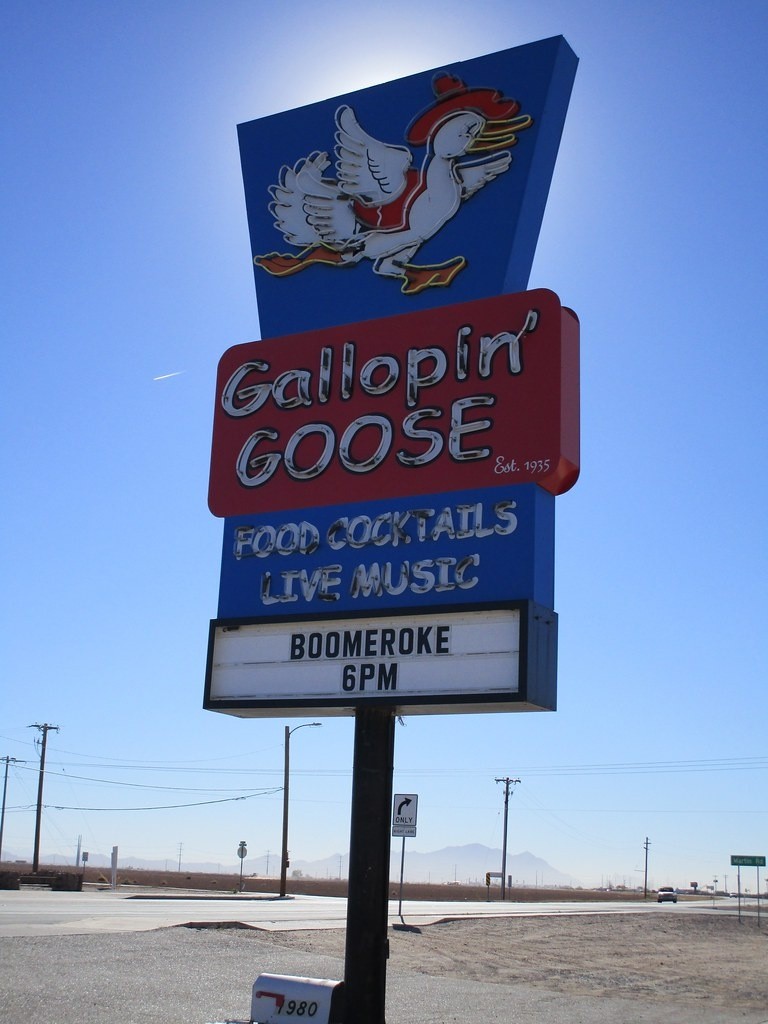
[278,722,324,896]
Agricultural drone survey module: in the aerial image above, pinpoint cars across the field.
[730,892,737,899]
[657,887,678,905]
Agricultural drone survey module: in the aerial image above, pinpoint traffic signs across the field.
[393,793,418,828]
